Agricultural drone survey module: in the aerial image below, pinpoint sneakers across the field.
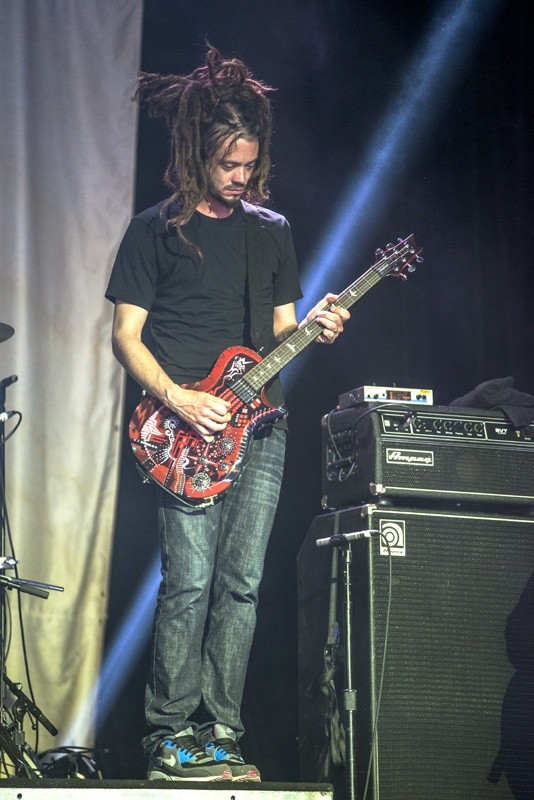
[146,727,233,781]
[196,724,262,783]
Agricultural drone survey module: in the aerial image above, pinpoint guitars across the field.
[126,231,427,505]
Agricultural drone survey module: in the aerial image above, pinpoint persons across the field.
[105,44,351,782]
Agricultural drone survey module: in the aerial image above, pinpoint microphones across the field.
[315,529,379,550]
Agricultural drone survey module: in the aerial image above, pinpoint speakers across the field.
[295,505,534,800]
[318,404,534,511]
